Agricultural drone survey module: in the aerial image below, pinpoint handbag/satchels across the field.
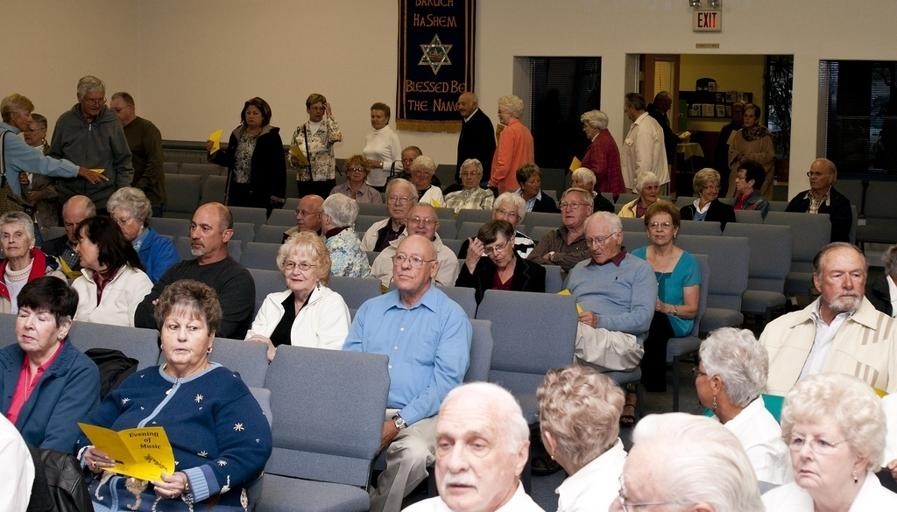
[384,175,399,191]
[0,174,34,216]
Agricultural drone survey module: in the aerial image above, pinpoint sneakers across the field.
[529,456,562,477]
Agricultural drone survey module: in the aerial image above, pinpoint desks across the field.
[676,142,704,161]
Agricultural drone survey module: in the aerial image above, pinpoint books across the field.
[76,422,175,483]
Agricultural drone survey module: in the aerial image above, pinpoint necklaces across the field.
[646,252,676,287]
[24,367,29,403]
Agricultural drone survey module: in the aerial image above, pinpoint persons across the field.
[688,326,787,486]
[394,382,548,512]
[439,162,778,431]
[534,364,630,512]
[755,240,895,465]
[784,156,854,244]
[76,280,273,511]
[361,103,403,195]
[576,89,686,204]
[208,97,286,211]
[2,75,255,342]
[288,92,343,201]
[759,376,897,511]
[452,93,533,198]
[0,277,101,511]
[223,149,473,511]
[608,410,766,512]
[717,102,775,202]
[867,246,896,318]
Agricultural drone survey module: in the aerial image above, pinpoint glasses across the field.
[350,167,364,173]
[483,240,508,256]
[703,185,721,191]
[560,201,590,211]
[617,474,671,511]
[84,95,107,106]
[691,367,708,379]
[409,217,436,226]
[648,221,674,229]
[807,171,824,177]
[294,209,320,217]
[789,433,848,455]
[393,253,435,266]
[285,260,319,271]
[585,232,617,247]
[387,194,415,204]
[495,206,517,219]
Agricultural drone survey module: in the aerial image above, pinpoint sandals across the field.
[620,403,635,426]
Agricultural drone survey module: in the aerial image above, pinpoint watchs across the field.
[379,160,383,169]
[671,304,678,317]
[548,251,555,262]
[182,473,190,494]
[391,414,407,430]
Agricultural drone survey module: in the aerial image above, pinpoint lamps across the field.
[705,1,723,11]
[688,0,703,11]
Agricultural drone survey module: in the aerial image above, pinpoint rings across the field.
[173,490,178,498]
[91,461,97,469]
